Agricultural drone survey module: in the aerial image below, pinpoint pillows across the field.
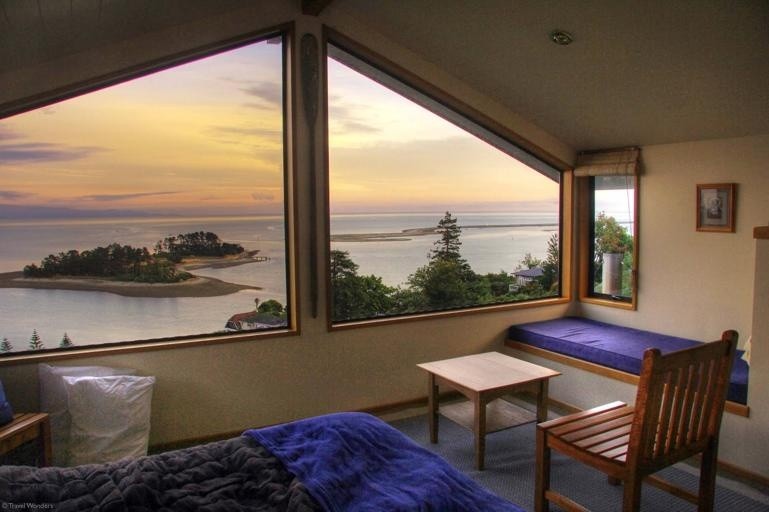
[39,362,136,467]
[63,375,156,464]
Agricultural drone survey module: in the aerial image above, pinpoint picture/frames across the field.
[695,182,736,232]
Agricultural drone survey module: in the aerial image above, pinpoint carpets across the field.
[387,399,769,511]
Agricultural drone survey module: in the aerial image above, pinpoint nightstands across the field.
[1,410,53,468]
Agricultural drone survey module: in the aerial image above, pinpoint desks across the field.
[416,352,561,470]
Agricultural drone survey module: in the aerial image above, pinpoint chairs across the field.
[534,329,739,511]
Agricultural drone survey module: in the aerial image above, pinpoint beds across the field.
[503,315,747,483]
[0,412,523,511]
